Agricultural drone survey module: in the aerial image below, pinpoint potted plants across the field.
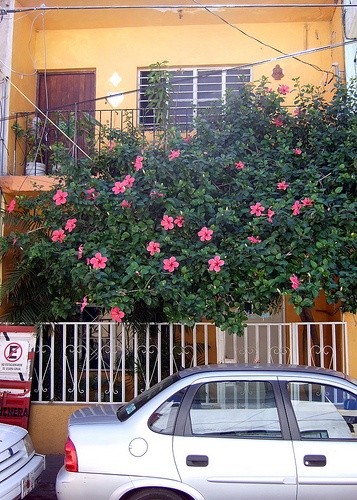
[11,123,72,176]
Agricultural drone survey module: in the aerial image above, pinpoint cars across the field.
[0,420,46,500]
[55,362,357,500]
